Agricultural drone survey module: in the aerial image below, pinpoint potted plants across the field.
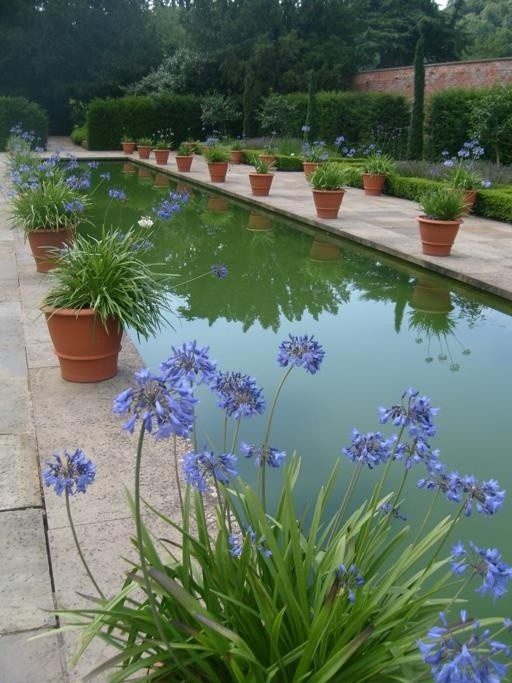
[248,154,274,197]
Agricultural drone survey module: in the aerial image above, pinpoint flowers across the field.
[300,271,352,302]
[298,125,358,163]
[444,135,493,187]
[202,224,233,245]
[414,330,481,372]
[37,337,508,683]
[181,132,245,162]
[358,140,381,160]
[241,259,282,292]
[101,186,226,277]
[264,129,279,143]
[4,122,125,211]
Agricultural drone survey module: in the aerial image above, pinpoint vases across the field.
[121,163,135,174]
[38,285,125,382]
[259,152,276,169]
[311,186,349,220]
[208,193,236,218]
[155,175,172,190]
[417,212,462,257]
[178,182,199,196]
[243,209,278,234]
[406,277,459,318]
[303,240,344,264]
[209,161,228,184]
[136,168,153,181]
[26,217,74,278]
[361,171,384,197]
[121,125,195,174]
